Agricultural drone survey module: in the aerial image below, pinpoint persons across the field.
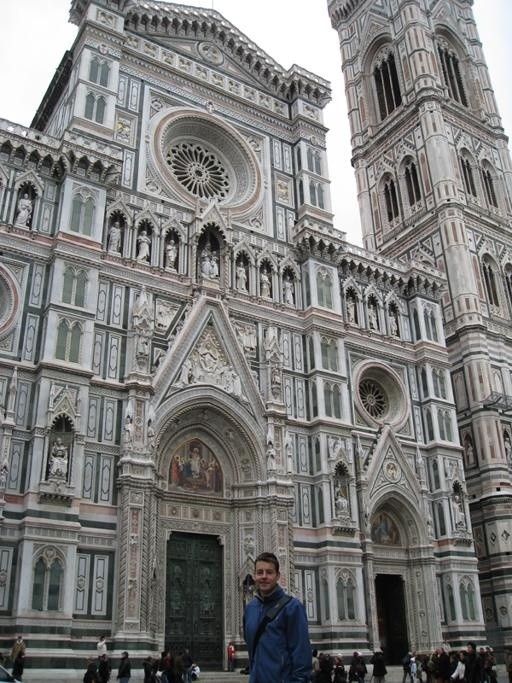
[236,262,248,291]
[312,641,512,683]
[346,438,352,461]
[241,551,312,683]
[260,269,271,298]
[84,656,97,683]
[467,443,474,464]
[117,651,131,683]
[265,443,278,471]
[97,635,107,672]
[147,421,156,452]
[457,459,466,481]
[136,231,151,261]
[287,444,293,472]
[108,221,123,252]
[283,275,295,304]
[6,635,29,667]
[99,654,108,683]
[11,650,26,682]
[443,456,451,477]
[174,446,223,493]
[143,648,201,682]
[334,480,348,512]
[504,437,512,460]
[50,437,69,478]
[123,418,134,450]
[452,495,466,527]
[201,242,219,277]
[367,305,379,330]
[388,312,398,335]
[14,193,33,229]
[328,436,335,458]
[226,642,235,672]
[165,238,177,268]
[346,296,356,322]
